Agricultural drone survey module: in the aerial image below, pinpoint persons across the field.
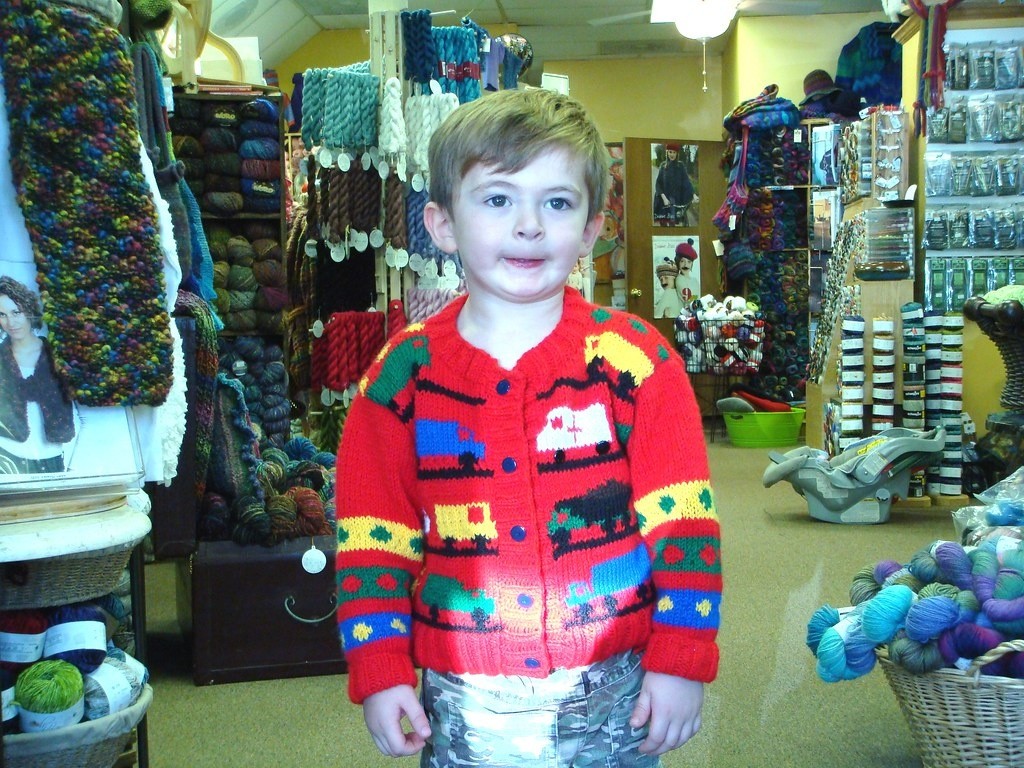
[336,86,722,768]
[655,243,700,318]
[0,276,76,474]
[653,144,694,227]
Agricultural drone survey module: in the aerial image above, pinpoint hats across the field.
[675,239,698,260]
[656,257,678,277]
[798,68,843,106]
[666,143,680,152]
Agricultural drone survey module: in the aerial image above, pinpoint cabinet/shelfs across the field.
[161,83,288,423]
[0,485,154,768]
[733,0,1024,519]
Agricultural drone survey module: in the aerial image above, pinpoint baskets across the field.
[0,536,145,611]
[0,682,154,768]
[873,639,1024,768]
